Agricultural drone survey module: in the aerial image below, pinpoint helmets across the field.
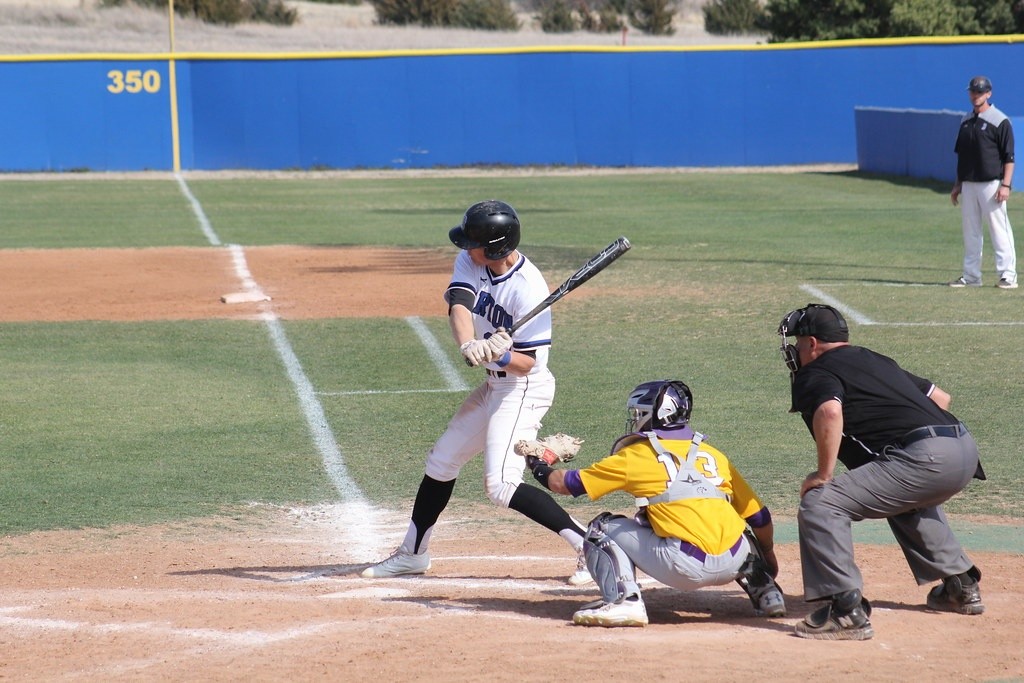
[778,303,849,342]
[449,201,520,260]
[628,379,693,432]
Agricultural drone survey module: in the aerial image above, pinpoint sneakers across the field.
[795,589,873,641]
[995,278,1018,289]
[746,572,786,618]
[949,276,982,288]
[360,545,431,577]
[567,545,594,585]
[573,592,649,627]
[927,578,984,615]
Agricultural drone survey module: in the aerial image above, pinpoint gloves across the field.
[460,327,513,366]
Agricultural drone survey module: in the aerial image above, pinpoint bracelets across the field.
[533,465,557,492]
[1001,184,1011,188]
[496,351,511,367]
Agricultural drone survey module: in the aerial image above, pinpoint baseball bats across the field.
[466,236,633,367]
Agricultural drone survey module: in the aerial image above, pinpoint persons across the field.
[526,379,786,626]
[949,77,1018,288]
[361,201,594,587]
[777,303,986,641]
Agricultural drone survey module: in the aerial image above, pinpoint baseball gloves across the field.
[514,431,580,464]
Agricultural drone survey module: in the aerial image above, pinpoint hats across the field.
[966,77,992,93]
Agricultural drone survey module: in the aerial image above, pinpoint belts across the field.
[680,535,743,563]
[486,368,506,377]
[899,422,967,448]
